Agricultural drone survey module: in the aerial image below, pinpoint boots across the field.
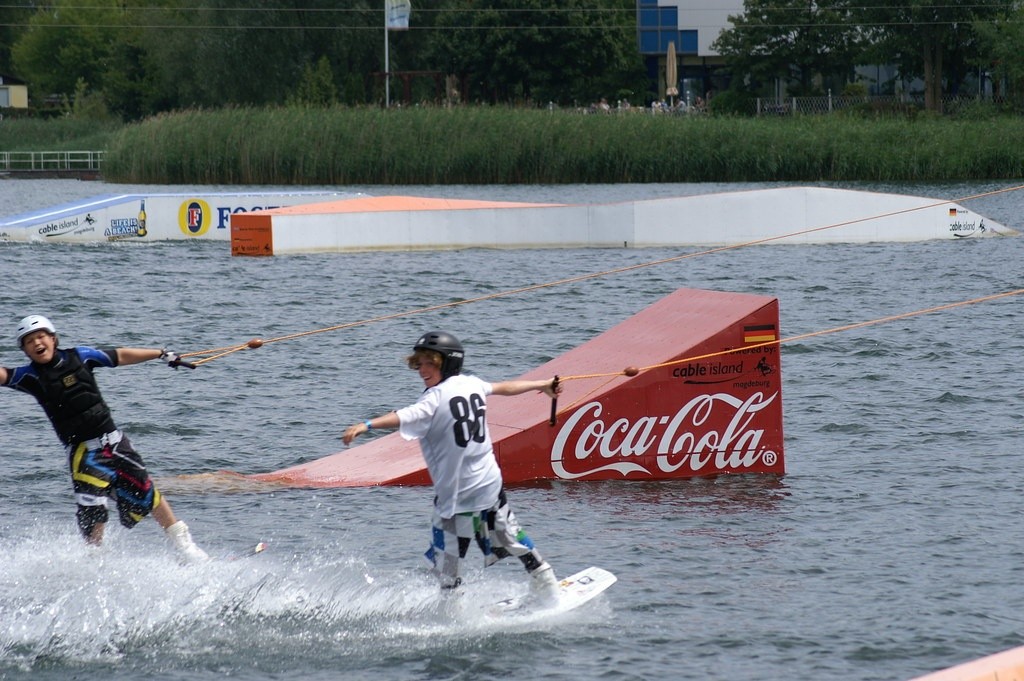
[166,520,208,562]
[531,563,560,603]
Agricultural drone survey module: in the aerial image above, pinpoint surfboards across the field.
[215,542,268,565]
[292,565,618,642]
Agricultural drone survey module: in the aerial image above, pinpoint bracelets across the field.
[364,421,372,431]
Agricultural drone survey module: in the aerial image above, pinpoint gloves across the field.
[158,350,181,368]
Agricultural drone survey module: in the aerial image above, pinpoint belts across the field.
[65,431,121,457]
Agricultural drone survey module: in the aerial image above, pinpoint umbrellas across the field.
[666,41,678,112]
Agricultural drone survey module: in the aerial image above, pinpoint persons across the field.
[341,333,562,607]
[597,98,629,114]
[649,95,709,119]
[0,315,213,563]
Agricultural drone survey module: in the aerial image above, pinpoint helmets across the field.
[413,331,464,376]
[16,314,55,349]
